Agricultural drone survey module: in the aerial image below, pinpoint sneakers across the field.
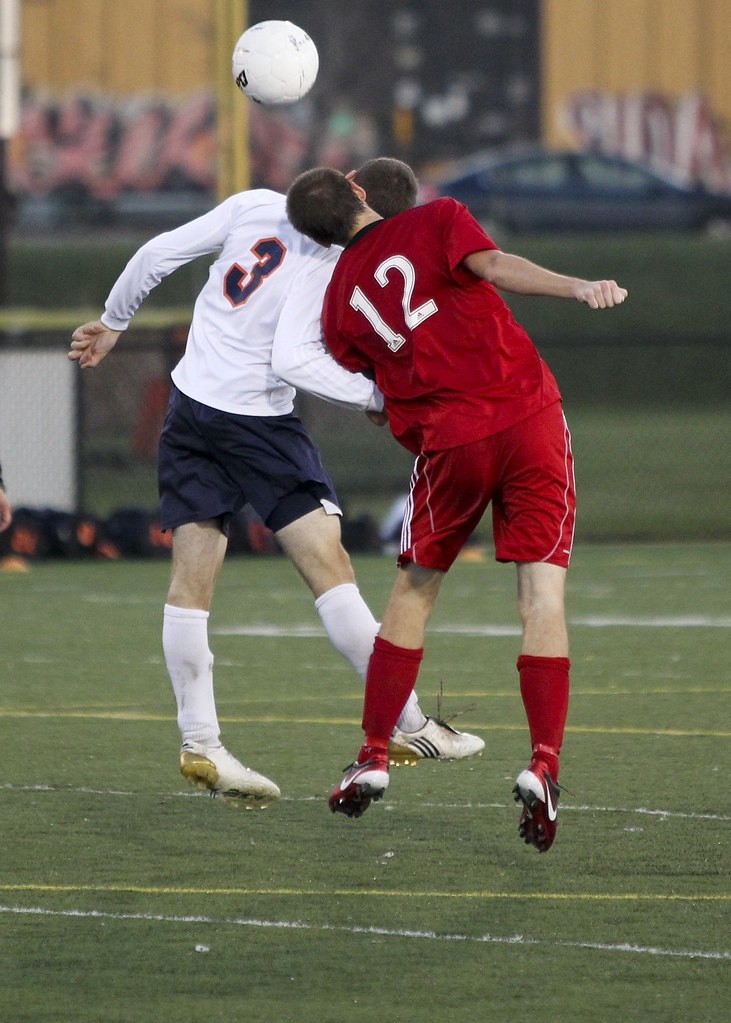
[511,761,576,853]
[180,752,280,810]
[389,681,486,767]
[327,759,391,820]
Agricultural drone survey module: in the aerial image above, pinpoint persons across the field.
[69,156,487,812]
[287,167,628,855]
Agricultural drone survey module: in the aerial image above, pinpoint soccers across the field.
[232,18,321,108]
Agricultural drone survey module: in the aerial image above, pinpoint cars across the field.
[414,152,731,240]
